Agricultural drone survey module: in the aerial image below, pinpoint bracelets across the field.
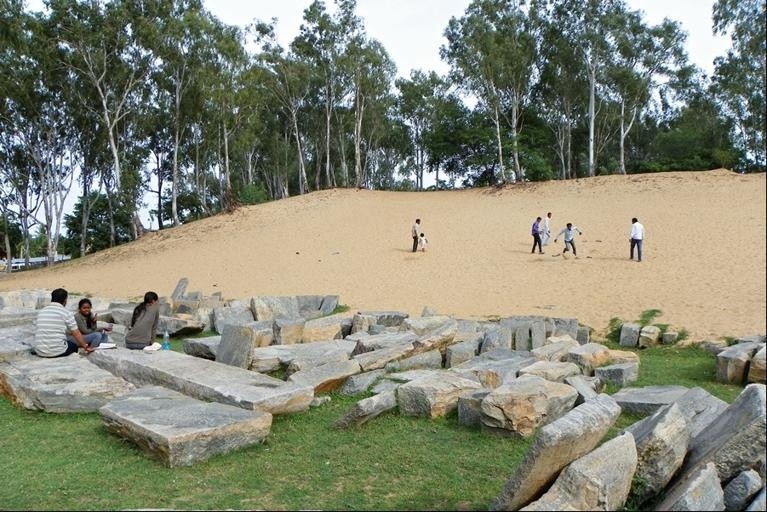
[84,344,88,351]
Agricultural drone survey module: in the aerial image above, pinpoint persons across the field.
[531,217,544,254]
[554,223,582,256]
[65,298,112,348]
[419,233,428,252]
[540,212,551,246]
[629,217,645,262]
[124,292,159,350]
[33,288,95,358]
[411,219,420,252]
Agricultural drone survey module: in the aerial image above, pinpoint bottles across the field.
[162,329,171,351]
